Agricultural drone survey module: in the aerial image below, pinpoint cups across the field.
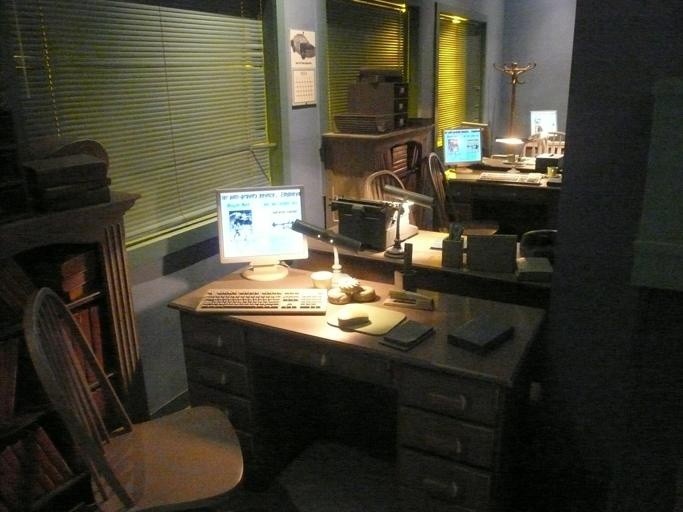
[547,166,558,177]
[309,270,332,290]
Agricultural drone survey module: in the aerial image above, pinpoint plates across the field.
[543,173,560,178]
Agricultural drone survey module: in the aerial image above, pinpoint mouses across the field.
[337,311,369,327]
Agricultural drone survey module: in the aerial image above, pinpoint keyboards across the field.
[195,288,328,316]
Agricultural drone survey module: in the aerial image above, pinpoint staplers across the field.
[383,290,434,311]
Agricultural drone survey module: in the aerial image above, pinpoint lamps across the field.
[291,219,377,304]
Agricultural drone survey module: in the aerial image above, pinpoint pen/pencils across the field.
[447,223,465,241]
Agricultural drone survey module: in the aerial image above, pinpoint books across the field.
[383,319,432,346]
[446,315,516,355]
[0,242,109,510]
[377,325,435,352]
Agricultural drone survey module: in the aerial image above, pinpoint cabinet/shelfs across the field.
[318,118,436,231]
[1,190,150,511]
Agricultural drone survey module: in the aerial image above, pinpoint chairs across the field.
[363,170,416,228]
[425,152,499,235]
[22,285,244,511]
[522,131,565,158]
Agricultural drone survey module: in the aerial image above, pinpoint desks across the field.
[444,162,560,229]
[167,267,546,511]
[289,225,549,308]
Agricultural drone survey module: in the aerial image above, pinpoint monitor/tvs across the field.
[528,110,557,143]
[441,127,483,173]
[215,185,309,281]
